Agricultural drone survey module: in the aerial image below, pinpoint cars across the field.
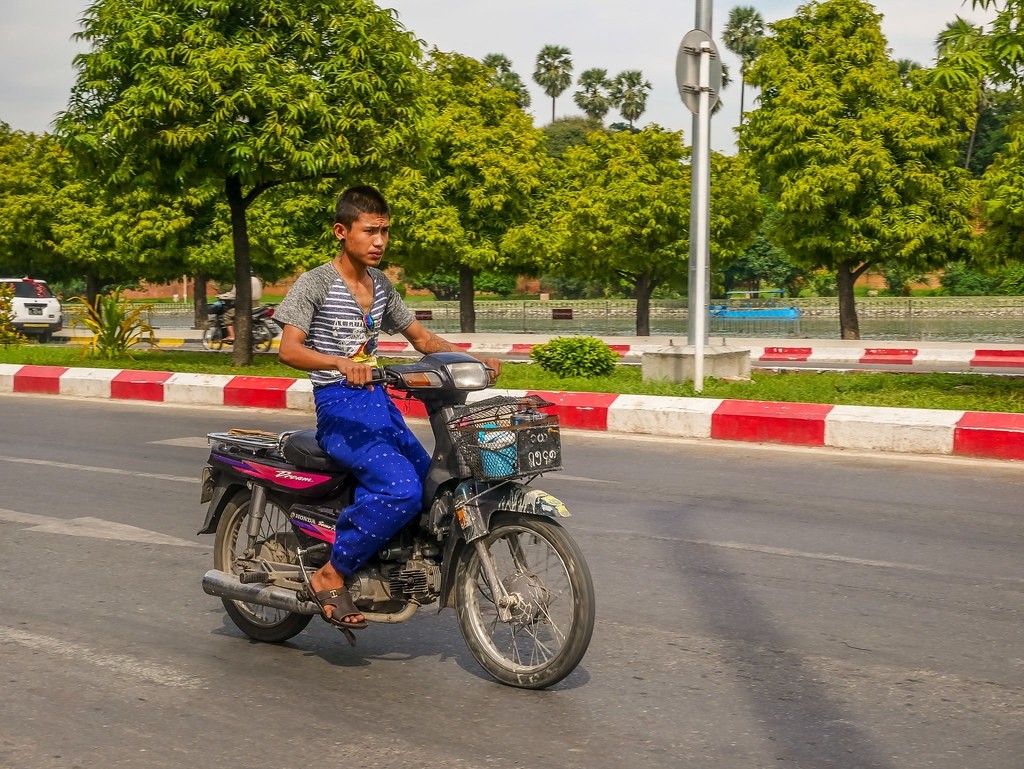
[0,277,65,343]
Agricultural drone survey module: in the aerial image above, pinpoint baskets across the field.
[446,396,563,478]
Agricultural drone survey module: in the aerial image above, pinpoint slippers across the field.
[306,583,368,628]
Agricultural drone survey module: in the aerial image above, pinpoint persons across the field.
[218,269,261,341]
[271,187,501,628]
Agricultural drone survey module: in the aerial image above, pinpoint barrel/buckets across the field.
[477,424,517,477]
[510,413,548,434]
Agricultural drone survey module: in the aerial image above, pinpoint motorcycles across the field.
[196,352,597,689]
[202,285,280,354]
